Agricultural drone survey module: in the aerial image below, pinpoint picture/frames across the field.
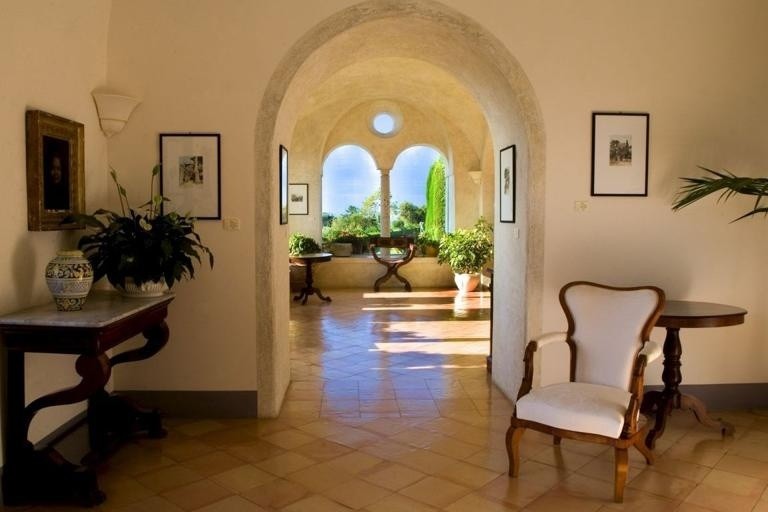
[588,110,649,197]
[158,132,222,221]
[499,143,517,225]
[23,106,88,233]
[278,142,290,226]
[288,182,310,216]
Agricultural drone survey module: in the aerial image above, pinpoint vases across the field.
[43,249,94,314]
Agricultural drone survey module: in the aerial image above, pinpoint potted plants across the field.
[288,231,322,294]
[435,216,494,293]
[55,163,215,298]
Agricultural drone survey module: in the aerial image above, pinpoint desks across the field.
[289,252,334,307]
[640,298,748,451]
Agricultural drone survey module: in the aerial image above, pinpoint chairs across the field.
[503,281,665,505]
[367,233,418,292]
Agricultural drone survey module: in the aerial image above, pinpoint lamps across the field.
[468,169,482,186]
[90,90,143,140]
